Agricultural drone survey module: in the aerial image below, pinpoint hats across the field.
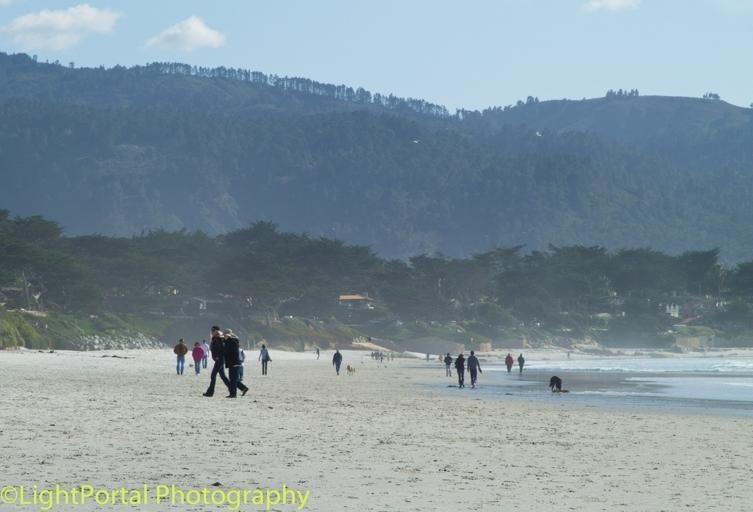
[222,327,233,339]
[212,326,220,331]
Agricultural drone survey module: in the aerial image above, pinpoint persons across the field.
[370,351,389,363]
[256,344,272,376]
[190,342,203,374]
[174,337,186,375]
[504,353,513,372]
[455,353,465,388]
[238,346,244,383]
[467,350,482,389]
[332,347,342,376]
[517,353,524,372]
[315,346,320,359]
[219,328,248,398]
[443,352,451,376]
[199,339,209,369]
[201,325,230,396]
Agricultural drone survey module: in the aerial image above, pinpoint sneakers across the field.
[201,386,248,398]
